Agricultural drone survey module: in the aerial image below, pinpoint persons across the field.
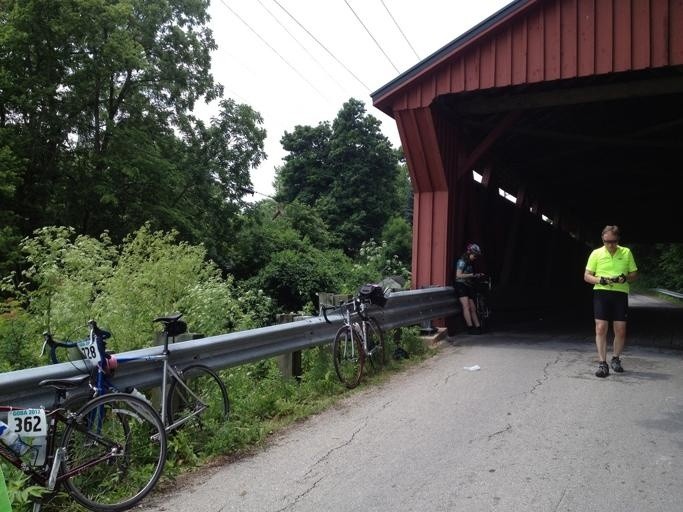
[584,225,638,377]
[455,243,486,330]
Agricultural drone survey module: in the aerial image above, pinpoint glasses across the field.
[603,240,617,243]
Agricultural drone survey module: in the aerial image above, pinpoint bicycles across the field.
[0,379,166,511]
[320,293,384,390]
[40,314,230,503]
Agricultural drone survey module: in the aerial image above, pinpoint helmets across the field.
[467,244,481,256]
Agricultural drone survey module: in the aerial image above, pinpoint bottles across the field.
[0,421,32,456]
[353,321,364,341]
[125,385,152,407]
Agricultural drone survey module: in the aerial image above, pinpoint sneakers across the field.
[611,357,623,372]
[596,362,608,377]
[468,326,481,335]
